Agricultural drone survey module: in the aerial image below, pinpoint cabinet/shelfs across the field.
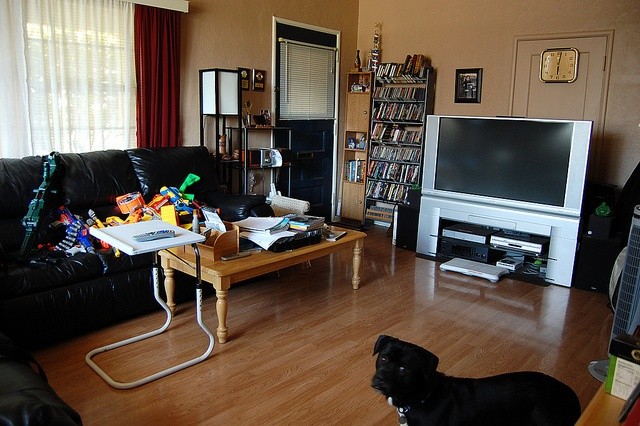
[363,67,436,228]
[339,71,372,224]
[228,126,277,195]
[415,194,582,289]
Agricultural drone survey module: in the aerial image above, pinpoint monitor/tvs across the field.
[420,114,594,218]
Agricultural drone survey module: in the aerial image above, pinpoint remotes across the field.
[131,229,177,242]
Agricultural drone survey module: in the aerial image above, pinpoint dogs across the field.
[371,334,582,426]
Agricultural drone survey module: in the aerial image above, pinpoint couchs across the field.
[0,146,274,352]
[0,344,83,426]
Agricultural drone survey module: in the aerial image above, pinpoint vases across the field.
[354,49,362,69]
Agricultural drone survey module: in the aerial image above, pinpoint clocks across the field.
[538,46,579,84]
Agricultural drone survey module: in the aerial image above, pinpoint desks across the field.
[571,368,630,424]
[84,219,213,391]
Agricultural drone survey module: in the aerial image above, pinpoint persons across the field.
[463,75,473,98]
[359,136,366,150]
[348,139,355,149]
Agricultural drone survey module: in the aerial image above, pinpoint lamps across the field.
[199,68,243,198]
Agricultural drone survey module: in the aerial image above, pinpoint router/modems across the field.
[496,256,524,272]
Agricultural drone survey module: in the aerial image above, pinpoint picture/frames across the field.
[454,68,483,103]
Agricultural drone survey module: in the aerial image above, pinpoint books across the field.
[376,102,424,122]
[279,212,326,232]
[230,217,290,235]
[370,123,423,145]
[367,161,419,184]
[364,181,413,205]
[372,87,425,101]
[344,160,365,183]
[376,54,430,84]
[367,206,393,219]
[371,146,420,163]
